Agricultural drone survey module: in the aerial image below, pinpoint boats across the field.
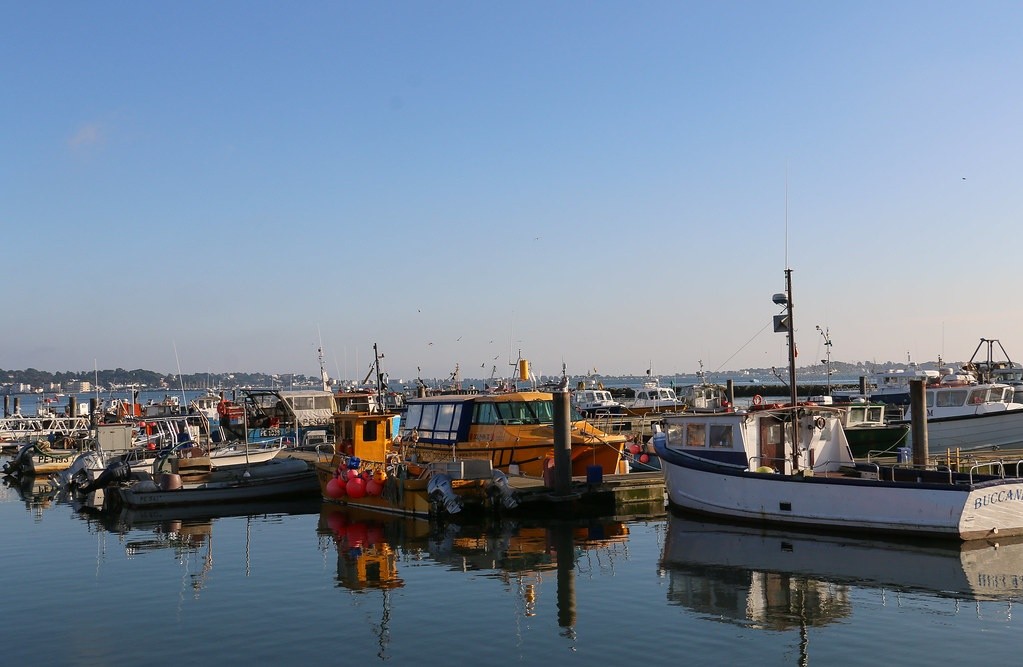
[649,179,1023,540]
[658,504,1023,667]
[0,322,1022,519]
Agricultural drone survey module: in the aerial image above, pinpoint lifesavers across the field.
[784,401,818,407]
[749,404,779,410]
[338,439,353,456]
[753,395,762,406]
[217,402,226,415]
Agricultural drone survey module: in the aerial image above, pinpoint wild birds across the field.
[417,308,500,368]
